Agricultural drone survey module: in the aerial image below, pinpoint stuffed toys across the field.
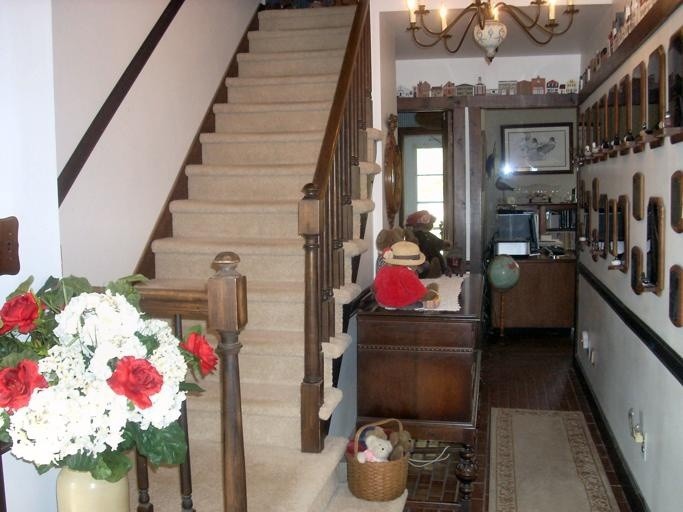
[374,227,406,275]
[346,417,416,467]
[371,242,440,310]
[404,210,454,279]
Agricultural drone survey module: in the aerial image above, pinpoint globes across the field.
[487,254,521,346]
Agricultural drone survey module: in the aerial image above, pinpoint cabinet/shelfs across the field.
[483,248,576,342]
[354,272,489,511]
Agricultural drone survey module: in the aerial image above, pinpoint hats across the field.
[382,241,425,267]
[406,209,436,225]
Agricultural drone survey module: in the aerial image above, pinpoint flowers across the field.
[0,260,225,489]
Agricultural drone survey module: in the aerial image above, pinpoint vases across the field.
[49,456,132,511]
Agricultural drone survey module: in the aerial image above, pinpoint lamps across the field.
[399,0,580,66]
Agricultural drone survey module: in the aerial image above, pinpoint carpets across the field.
[483,400,623,512]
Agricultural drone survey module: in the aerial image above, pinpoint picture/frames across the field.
[496,120,574,177]
[380,111,405,230]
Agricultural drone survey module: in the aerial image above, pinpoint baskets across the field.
[345,418,411,502]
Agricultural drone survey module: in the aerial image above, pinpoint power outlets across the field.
[639,431,648,463]
